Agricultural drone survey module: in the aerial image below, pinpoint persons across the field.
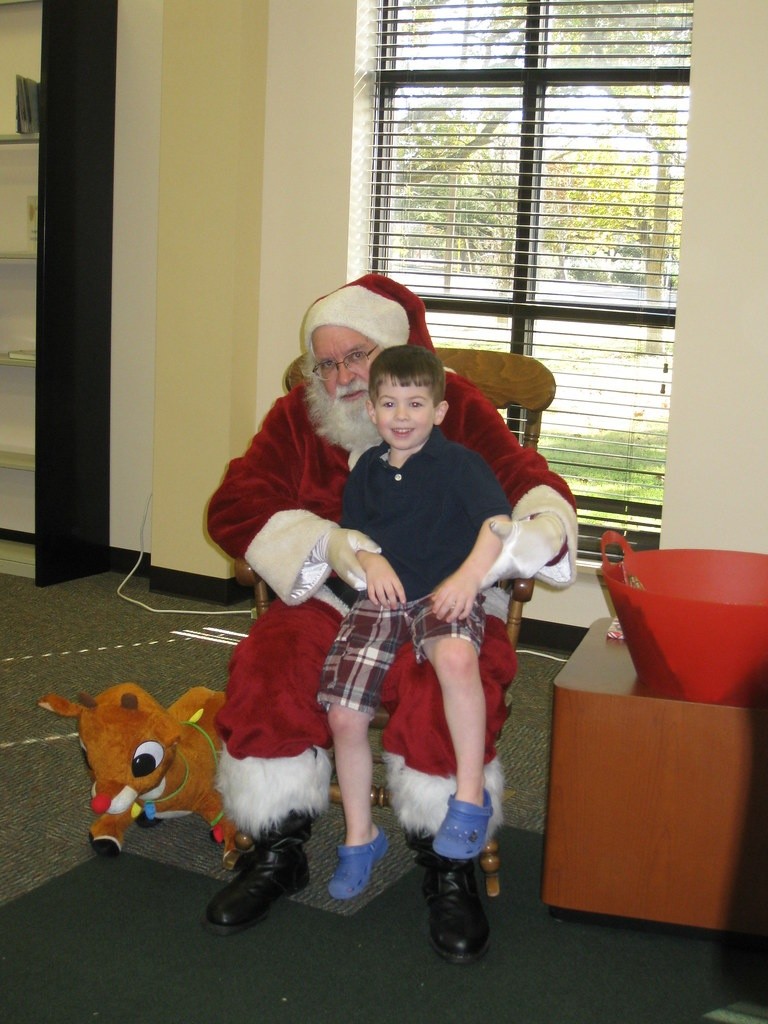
[316,342,516,900]
[196,274,580,970]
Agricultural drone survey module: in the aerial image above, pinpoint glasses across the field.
[312,344,380,381]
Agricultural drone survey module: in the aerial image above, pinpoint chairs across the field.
[222,347,555,899]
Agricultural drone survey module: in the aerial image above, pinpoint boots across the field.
[207,808,312,936]
[404,827,490,961]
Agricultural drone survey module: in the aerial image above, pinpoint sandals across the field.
[327,829,389,900]
[432,787,493,859]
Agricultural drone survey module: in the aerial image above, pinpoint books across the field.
[13,74,40,135]
[8,350,37,361]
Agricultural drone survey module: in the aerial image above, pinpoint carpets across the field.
[0,819,768,1024]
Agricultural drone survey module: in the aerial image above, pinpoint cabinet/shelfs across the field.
[1,134,114,585]
[538,616,768,938]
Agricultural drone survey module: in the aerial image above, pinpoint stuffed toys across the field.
[36,681,246,871]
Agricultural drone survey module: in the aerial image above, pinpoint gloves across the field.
[322,528,382,591]
[477,513,566,593]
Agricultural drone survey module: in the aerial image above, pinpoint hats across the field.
[303,272,434,356]
